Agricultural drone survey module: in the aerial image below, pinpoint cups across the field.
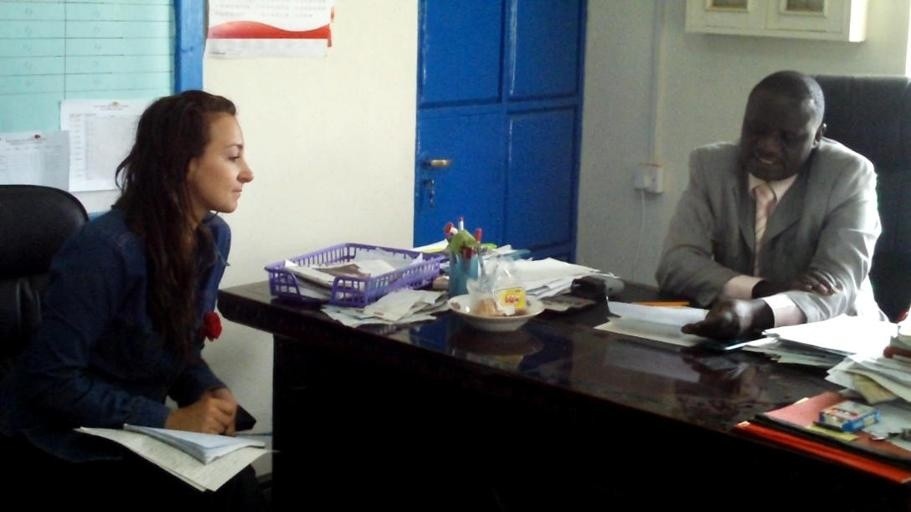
[449,255,480,293]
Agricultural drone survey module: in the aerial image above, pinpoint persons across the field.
[655,68,883,347]
[0,89,265,509]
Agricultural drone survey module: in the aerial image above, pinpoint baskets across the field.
[265,244,441,308]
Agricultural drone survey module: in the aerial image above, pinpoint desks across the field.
[218,276,911,512]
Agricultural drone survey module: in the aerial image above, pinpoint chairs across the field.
[0,183,92,370]
[813,74,911,322]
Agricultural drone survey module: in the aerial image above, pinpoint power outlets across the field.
[636,164,664,195]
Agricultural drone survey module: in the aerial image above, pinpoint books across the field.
[120,415,267,462]
[604,299,910,489]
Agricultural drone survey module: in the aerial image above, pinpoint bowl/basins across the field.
[447,293,546,333]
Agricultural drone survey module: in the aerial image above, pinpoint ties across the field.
[755,183,775,277]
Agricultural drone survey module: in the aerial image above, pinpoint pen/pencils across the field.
[444,216,483,258]
[629,299,690,306]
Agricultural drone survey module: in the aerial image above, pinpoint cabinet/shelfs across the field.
[412,0,586,265]
[683,0,866,45]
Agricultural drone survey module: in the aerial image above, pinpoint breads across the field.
[475,298,529,317]
